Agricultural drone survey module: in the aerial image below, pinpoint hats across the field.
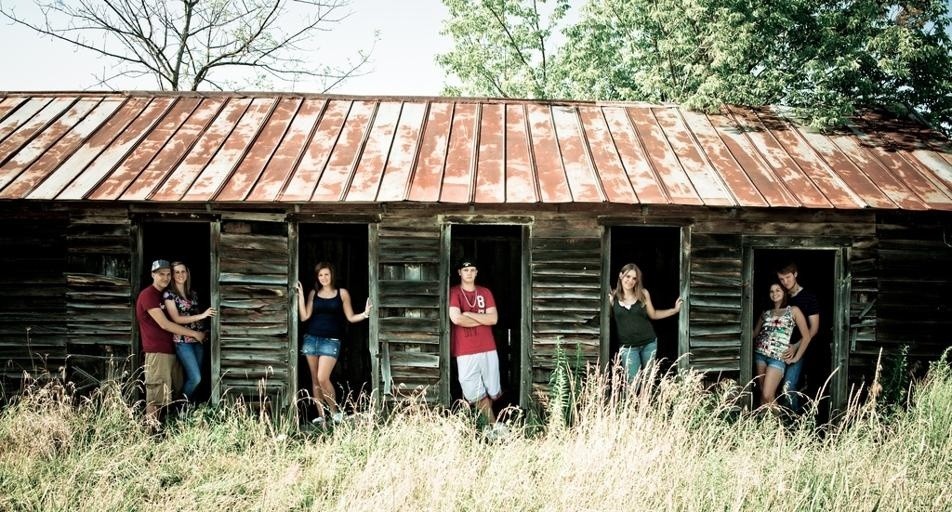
[458,258,477,269]
[152,260,170,273]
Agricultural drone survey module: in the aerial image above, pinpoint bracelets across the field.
[363,312,369,318]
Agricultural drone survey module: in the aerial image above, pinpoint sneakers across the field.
[481,422,506,443]
[332,413,340,423]
[312,417,323,424]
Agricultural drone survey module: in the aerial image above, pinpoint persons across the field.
[753,280,811,417]
[776,262,820,412]
[162,259,218,402]
[136,259,209,422]
[608,264,683,396]
[294,262,373,421]
[449,259,503,424]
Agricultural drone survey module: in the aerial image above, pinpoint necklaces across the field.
[461,286,478,308]
